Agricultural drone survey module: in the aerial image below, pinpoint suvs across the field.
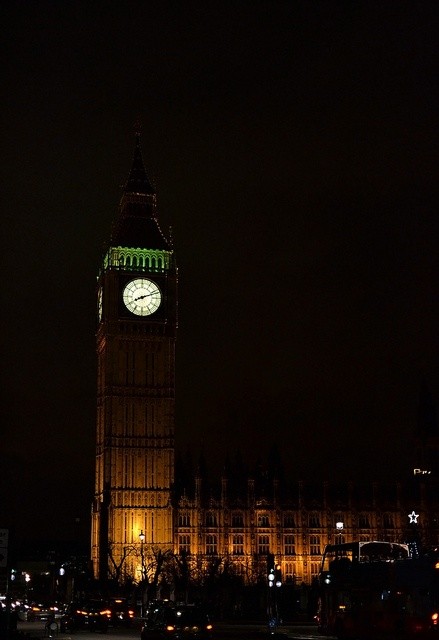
[107,597,135,628]
[58,598,111,632]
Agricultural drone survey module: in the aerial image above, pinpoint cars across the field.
[141,605,213,640]
[143,598,175,624]
[0,593,67,622]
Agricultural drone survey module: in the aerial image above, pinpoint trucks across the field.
[311,542,439,640]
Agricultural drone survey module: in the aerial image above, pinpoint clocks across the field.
[123,277,162,316]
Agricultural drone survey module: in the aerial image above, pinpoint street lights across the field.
[336,520,344,544]
[139,530,147,581]
